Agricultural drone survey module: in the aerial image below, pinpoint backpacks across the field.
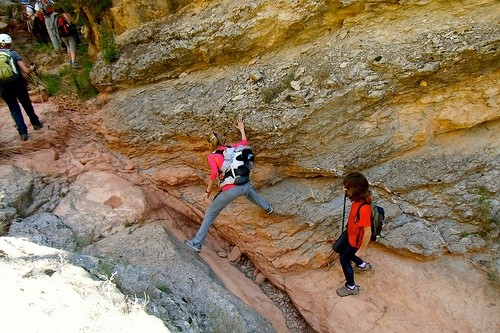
[26,5,36,14]
[40,0,55,15]
[0,49,22,80]
[212,145,255,188]
[54,14,72,38]
[353,202,385,242]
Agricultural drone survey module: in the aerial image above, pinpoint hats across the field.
[0,33,12,43]
[21,0,30,4]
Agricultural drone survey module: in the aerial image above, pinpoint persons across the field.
[21,0,80,69]
[332,171,373,297]
[184,117,273,253]
[0,33,44,141]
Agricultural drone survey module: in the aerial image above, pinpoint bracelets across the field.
[205,189,211,193]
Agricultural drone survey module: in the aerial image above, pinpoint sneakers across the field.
[184,240,202,251]
[352,261,371,273]
[336,282,360,297]
[265,200,273,215]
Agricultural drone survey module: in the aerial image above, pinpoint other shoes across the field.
[78,43,81,46]
[34,124,43,130]
[66,60,79,68]
[20,134,28,141]
[53,46,65,51]
